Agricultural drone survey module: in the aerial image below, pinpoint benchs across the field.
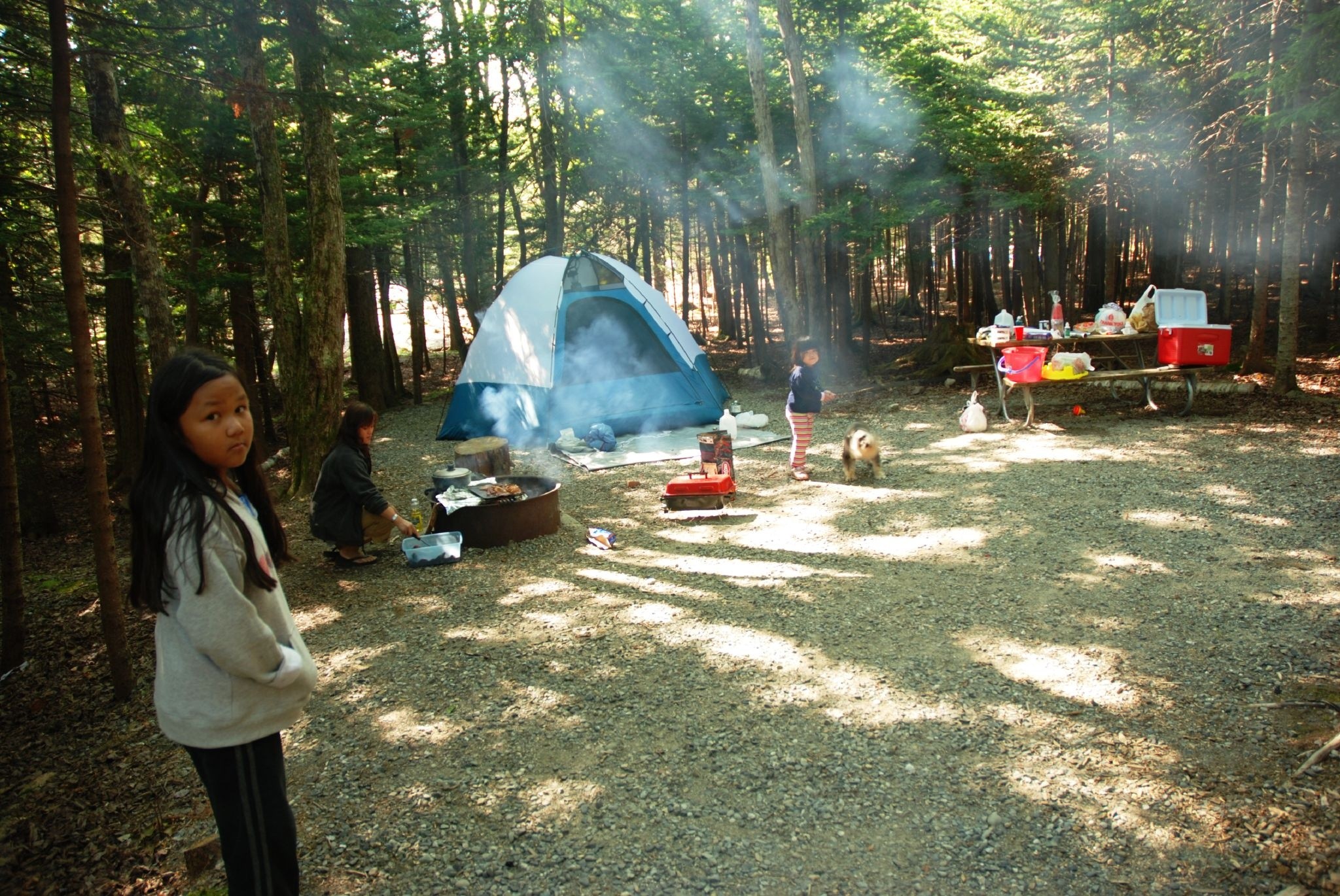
[1001,364,1214,428]
[953,354,1139,415]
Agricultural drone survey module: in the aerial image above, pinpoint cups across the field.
[1013,326,1024,341]
[1039,320,1049,330]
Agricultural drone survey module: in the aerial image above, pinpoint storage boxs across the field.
[661,492,735,511]
[696,429,736,483]
[1154,288,1233,366]
[402,531,463,568]
[1025,333,1053,340]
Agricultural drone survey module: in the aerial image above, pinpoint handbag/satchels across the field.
[959,391,987,432]
[1094,302,1126,335]
[1129,284,1156,316]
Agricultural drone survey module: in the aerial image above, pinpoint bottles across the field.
[730,400,741,417]
[410,498,424,534]
[719,408,737,440]
[1064,322,1070,338]
[994,309,1015,337]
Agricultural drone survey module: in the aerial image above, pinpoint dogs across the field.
[839,422,887,481]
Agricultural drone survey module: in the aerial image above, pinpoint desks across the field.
[966,332,1159,423]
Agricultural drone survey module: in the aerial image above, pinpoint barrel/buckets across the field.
[997,346,1048,383]
[422,474,561,549]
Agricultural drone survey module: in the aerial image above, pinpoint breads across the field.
[506,484,519,493]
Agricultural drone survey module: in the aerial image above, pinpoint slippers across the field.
[338,552,379,565]
[322,546,340,557]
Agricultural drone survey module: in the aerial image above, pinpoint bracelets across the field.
[391,514,399,523]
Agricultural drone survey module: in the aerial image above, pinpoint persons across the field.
[785,335,836,479]
[308,401,420,569]
[125,347,315,896]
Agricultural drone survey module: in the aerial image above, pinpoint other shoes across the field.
[790,466,808,480]
[791,463,814,473]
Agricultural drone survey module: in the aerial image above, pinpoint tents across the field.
[435,245,732,448]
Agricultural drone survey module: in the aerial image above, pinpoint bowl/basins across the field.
[431,464,471,492]
